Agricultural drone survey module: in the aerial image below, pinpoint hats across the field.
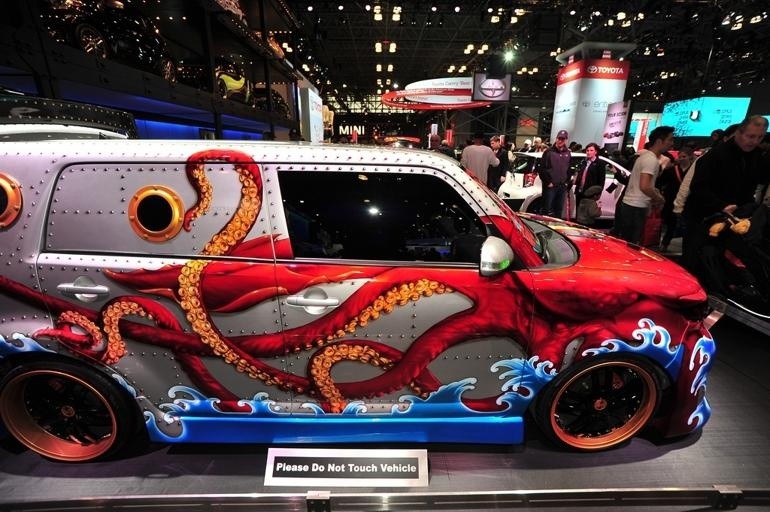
[558,130,568,139]
[584,186,602,197]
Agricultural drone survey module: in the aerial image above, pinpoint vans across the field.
[699,235,770,338]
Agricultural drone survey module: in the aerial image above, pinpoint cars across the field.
[496,147,636,228]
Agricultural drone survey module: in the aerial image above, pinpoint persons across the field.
[618,115,769,291]
[430,131,508,194]
[508,129,620,227]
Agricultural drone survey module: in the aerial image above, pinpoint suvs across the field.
[1,118,715,467]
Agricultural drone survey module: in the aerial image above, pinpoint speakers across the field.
[486,55,506,79]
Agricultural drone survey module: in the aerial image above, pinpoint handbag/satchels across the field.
[606,183,617,193]
[642,208,662,246]
